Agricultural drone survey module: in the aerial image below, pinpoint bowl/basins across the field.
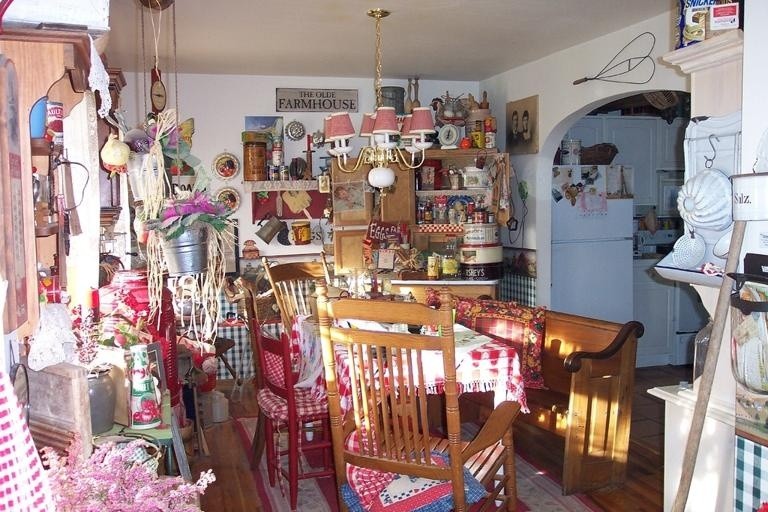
[712,227,733,259]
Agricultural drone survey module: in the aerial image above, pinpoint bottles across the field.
[417,195,493,224]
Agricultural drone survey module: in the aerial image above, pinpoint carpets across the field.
[232,414,600,511]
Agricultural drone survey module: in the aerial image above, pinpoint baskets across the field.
[643,91,679,110]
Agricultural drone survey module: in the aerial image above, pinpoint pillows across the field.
[451,294,550,393]
[345,418,405,510]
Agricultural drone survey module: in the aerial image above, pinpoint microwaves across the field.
[656,170,685,219]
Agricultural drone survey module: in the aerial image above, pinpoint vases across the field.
[158,226,209,277]
[177,174,195,189]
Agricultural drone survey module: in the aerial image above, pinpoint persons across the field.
[518,110,531,143]
[507,109,521,143]
[333,184,364,209]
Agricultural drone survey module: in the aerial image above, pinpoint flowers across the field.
[40,433,217,512]
[170,157,193,175]
[141,188,231,241]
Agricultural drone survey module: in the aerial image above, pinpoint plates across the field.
[215,188,240,213]
[212,152,240,180]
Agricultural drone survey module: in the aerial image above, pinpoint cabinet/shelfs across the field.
[377,146,498,303]
[2,27,125,342]
[643,28,766,509]
[571,114,689,215]
[631,257,710,368]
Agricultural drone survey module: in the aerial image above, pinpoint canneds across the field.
[427,257,438,276]
[484,132,495,149]
[291,221,311,245]
[561,140,582,165]
[272,151,284,166]
[278,165,288,180]
[483,116,497,132]
[267,165,279,181]
[272,136,283,151]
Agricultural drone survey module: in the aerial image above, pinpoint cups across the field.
[255,213,288,244]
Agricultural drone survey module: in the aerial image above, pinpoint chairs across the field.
[255,253,333,328]
[312,275,521,510]
[242,292,268,470]
[252,321,335,511]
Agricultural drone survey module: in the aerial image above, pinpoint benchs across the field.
[448,294,643,496]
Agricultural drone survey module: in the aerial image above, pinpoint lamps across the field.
[322,6,436,201]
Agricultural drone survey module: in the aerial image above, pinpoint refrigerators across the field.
[550,163,634,326]
